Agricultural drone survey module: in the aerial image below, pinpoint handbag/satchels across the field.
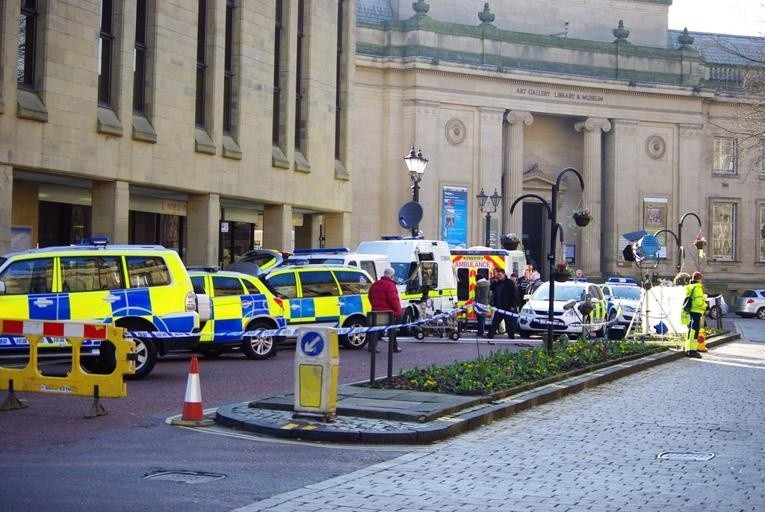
[683,297,692,311]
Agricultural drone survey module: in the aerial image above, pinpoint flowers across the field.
[550,263,576,278]
[567,207,594,222]
[694,233,705,241]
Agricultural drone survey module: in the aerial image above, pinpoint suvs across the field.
[0,235,213,380]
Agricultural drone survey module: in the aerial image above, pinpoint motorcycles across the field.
[413,285,459,340]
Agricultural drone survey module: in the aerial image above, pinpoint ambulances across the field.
[448,248,526,335]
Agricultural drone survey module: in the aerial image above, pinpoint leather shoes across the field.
[392,347,401,352]
[368,347,380,353]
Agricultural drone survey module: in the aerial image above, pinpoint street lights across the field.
[404,145,427,239]
[476,189,502,247]
[510,167,593,350]
[653,212,707,285]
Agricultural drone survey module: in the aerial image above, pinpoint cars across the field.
[601,276,644,339]
[518,278,605,338]
[703,293,728,320]
[182,244,381,357]
[734,288,765,320]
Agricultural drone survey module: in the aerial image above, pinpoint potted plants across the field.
[500,231,521,250]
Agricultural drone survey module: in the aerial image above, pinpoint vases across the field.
[575,216,590,226]
[695,241,706,249]
[551,272,570,282]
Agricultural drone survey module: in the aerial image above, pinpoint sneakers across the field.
[687,351,701,358]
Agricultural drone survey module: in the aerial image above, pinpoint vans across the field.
[359,234,458,336]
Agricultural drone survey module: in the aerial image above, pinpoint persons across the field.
[473,263,589,339]
[368,267,403,353]
[687,271,710,358]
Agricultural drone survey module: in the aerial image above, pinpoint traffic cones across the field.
[181,354,204,421]
